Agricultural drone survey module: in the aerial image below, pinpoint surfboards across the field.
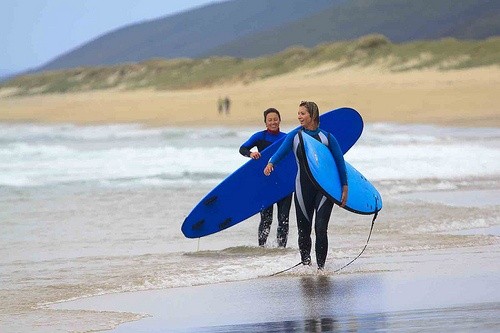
[293,130,383,216]
[180,106,364,238]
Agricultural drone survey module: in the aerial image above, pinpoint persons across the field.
[265,101,348,269]
[239,108,290,247]
